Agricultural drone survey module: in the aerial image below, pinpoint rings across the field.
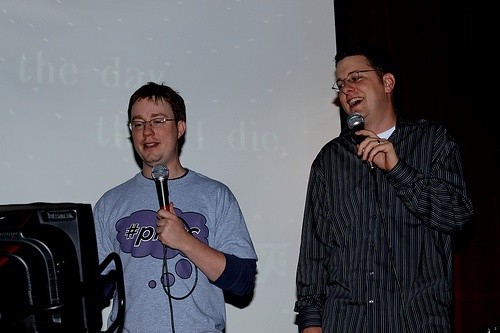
[377,140,380,144]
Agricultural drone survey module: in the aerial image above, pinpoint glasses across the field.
[332,70,379,93]
[127,118,174,131]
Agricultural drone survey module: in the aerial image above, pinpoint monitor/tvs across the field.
[0,202,102,333]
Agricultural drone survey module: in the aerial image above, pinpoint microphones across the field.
[151,163,169,213]
[347,113,375,167]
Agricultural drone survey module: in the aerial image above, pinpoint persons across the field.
[294,46,473,333]
[93,82,258,333]
